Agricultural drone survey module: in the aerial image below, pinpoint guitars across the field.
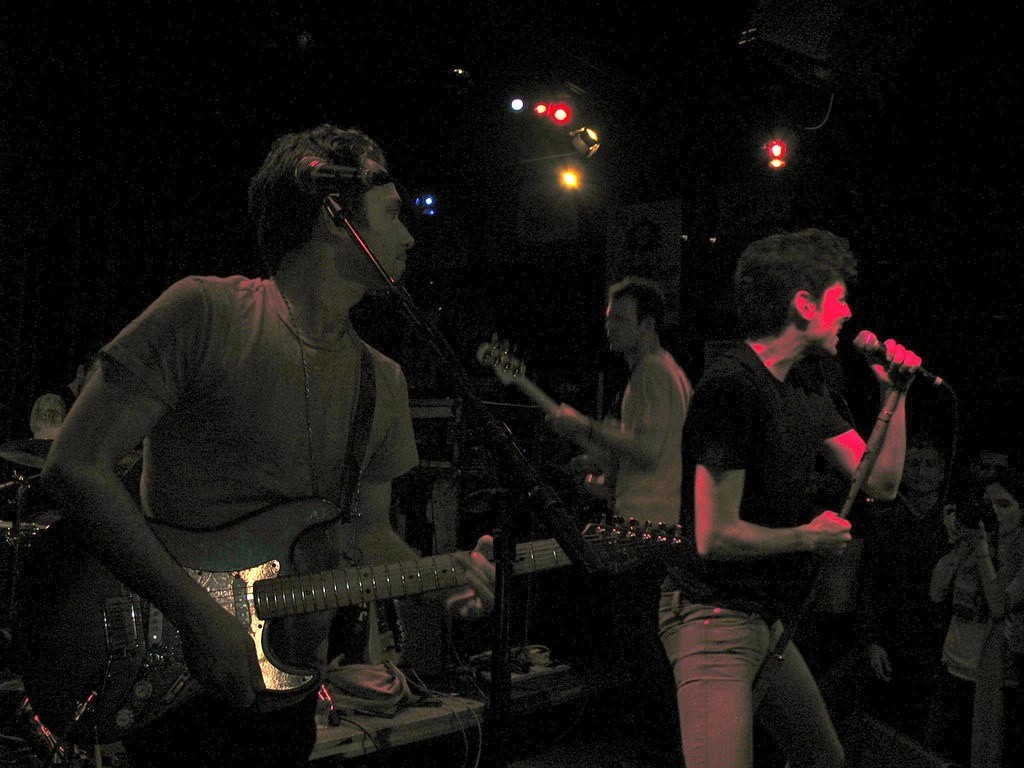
[475,333,618,474]
[95,493,682,731]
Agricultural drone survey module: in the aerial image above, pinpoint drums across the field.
[0,520,50,616]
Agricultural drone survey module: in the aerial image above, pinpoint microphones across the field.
[294,156,394,188]
[853,330,945,387]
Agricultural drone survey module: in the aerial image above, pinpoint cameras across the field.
[955,500,997,530]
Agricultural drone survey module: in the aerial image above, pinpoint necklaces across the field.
[277,277,364,565]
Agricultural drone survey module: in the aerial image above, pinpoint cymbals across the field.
[0,439,53,468]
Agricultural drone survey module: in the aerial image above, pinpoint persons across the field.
[20,339,106,523]
[544,278,693,768]
[656,228,922,767]
[856,432,956,742]
[41,127,494,768]
[921,465,1024,767]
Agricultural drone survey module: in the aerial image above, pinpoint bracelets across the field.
[588,422,593,440]
